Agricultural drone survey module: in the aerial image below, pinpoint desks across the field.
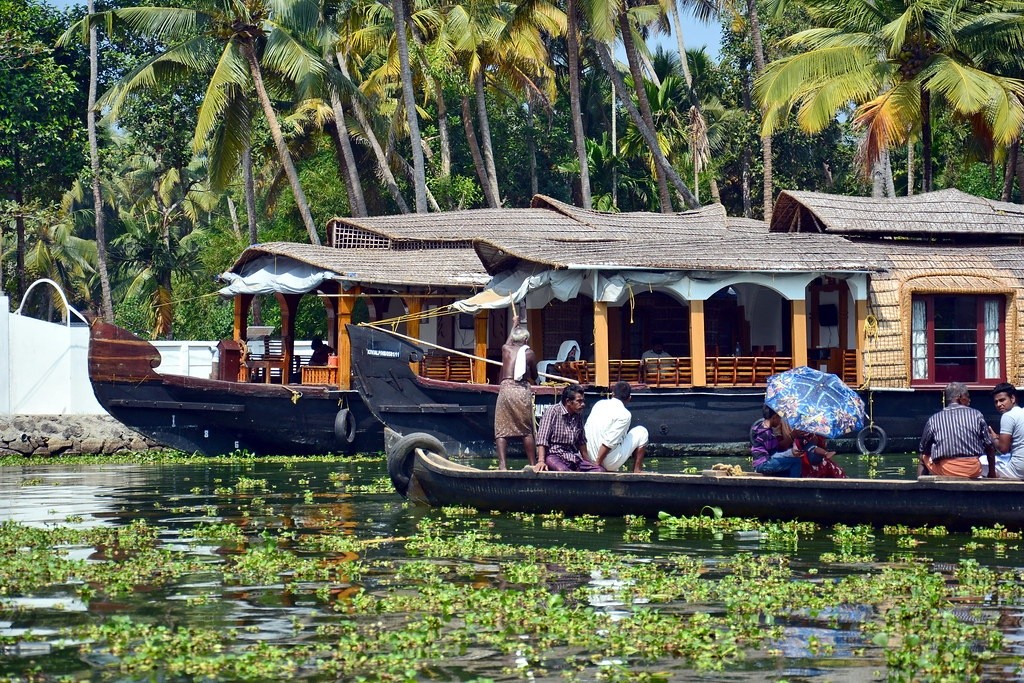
[245,360,284,384]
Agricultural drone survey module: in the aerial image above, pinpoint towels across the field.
[535,340,580,385]
[513,344,531,381]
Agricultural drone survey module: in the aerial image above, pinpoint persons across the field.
[751,403,837,478]
[917,382,996,478]
[583,381,649,472]
[493,315,538,470]
[534,384,607,472]
[978,383,1024,480]
[781,419,836,458]
[641,339,676,379]
[289,335,334,384]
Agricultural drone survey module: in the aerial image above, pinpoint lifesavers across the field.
[855,425,888,455]
[387,432,449,493]
[334,408,356,446]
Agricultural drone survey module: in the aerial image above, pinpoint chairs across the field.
[842,349,856,384]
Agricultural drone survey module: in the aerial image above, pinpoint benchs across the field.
[417,357,475,382]
[535,340,794,388]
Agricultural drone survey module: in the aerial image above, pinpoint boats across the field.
[88,187,1023,455]
[377,424,1024,531]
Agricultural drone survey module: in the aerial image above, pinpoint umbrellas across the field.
[764,366,865,439]
